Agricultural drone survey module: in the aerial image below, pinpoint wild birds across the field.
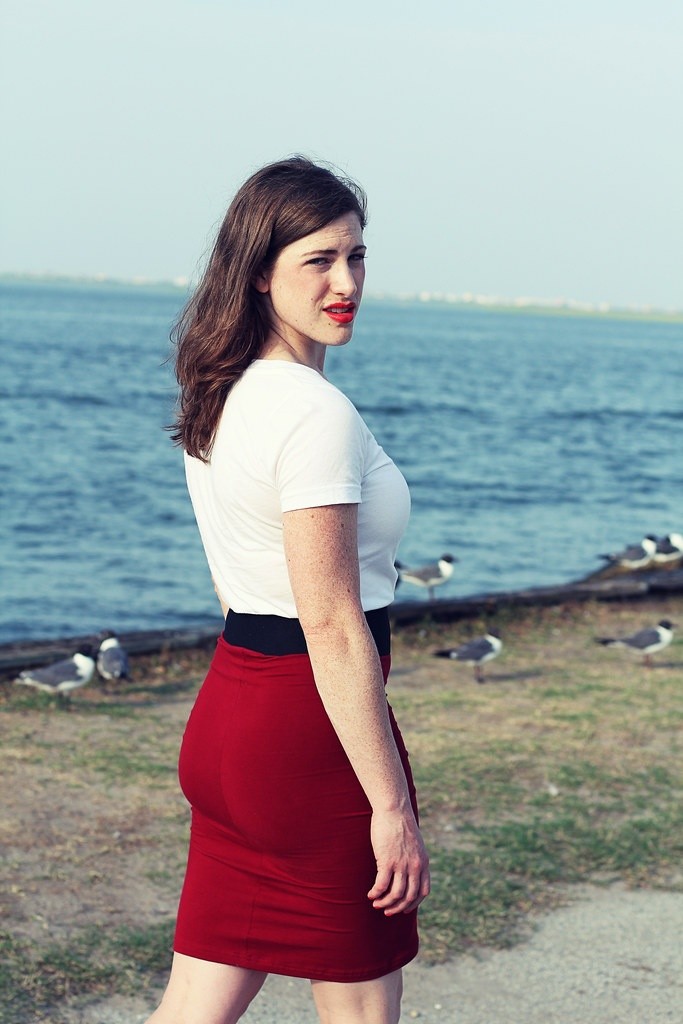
[393,552,461,602]
[430,627,503,684]
[594,531,683,572]
[11,643,95,713]
[96,628,134,696]
[598,618,680,668]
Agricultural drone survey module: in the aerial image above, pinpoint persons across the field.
[148,156,431,1024]
